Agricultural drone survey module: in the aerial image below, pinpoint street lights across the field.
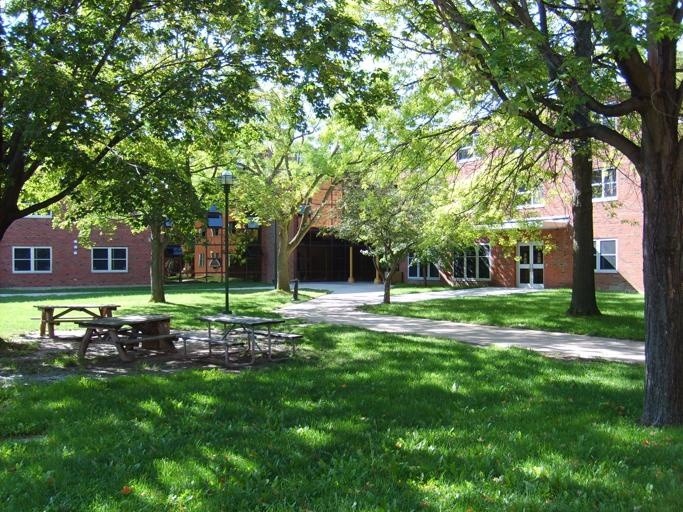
[222,167,234,315]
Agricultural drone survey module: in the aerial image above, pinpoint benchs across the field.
[30,303,303,369]
